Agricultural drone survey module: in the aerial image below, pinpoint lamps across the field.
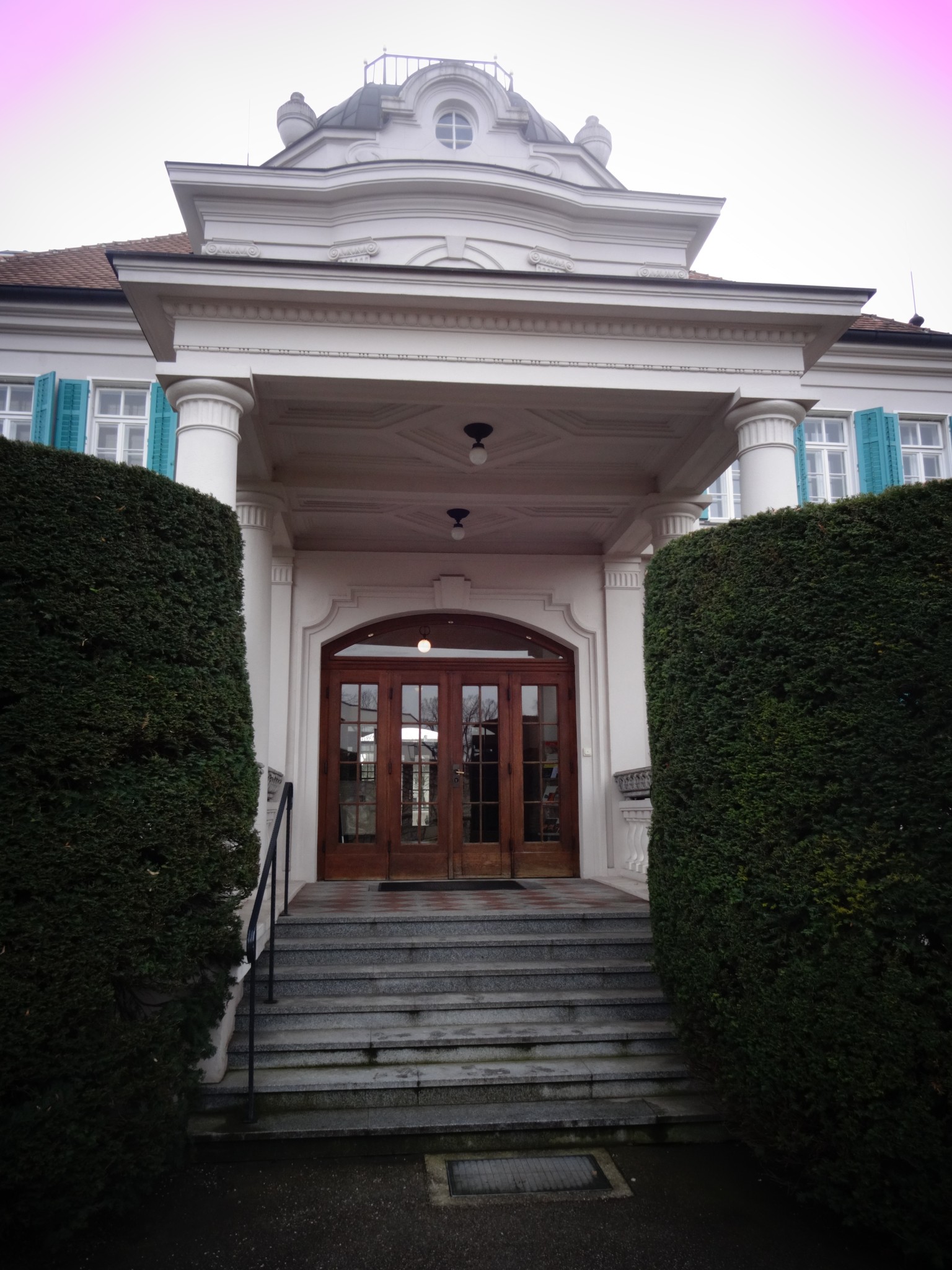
[447,507,470,540]
[463,421,493,466]
[417,626,432,653]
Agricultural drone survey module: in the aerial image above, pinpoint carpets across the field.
[378,881,528,891]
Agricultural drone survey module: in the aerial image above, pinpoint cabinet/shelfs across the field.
[538,763,560,842]
